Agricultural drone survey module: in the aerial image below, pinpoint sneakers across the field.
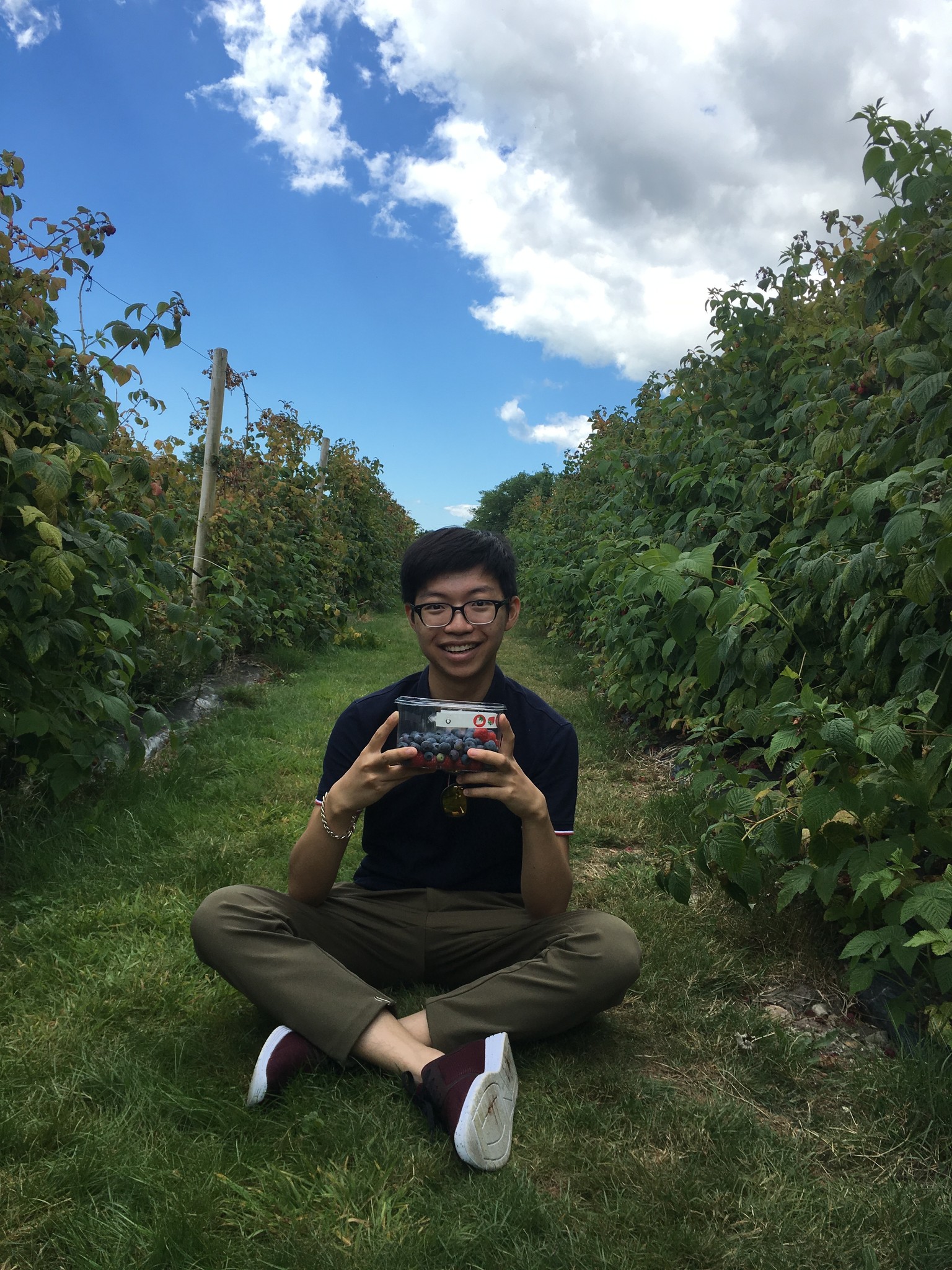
[248,1026,329,1111]
[401,1032,518,1170]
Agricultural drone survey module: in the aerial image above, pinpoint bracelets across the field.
[322,791,357,840]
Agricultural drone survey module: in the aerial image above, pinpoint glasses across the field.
[410,599,506,628]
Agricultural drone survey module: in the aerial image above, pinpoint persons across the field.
[193,527,644,1168]
